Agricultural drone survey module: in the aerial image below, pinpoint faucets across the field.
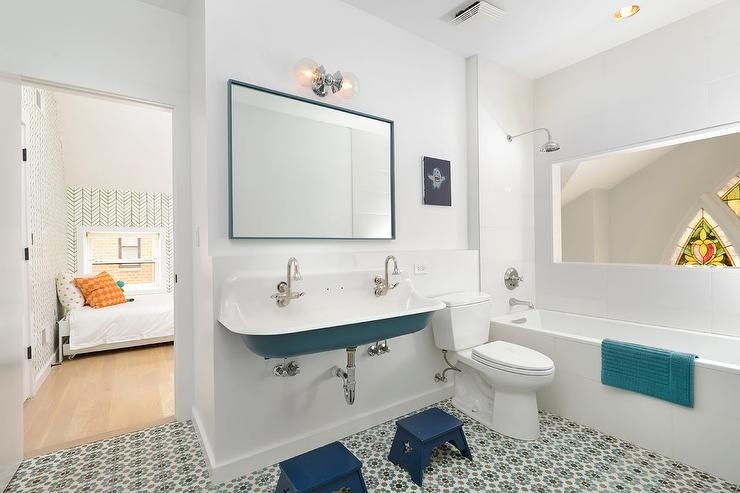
[508,296,539,312]
[376,254,403,298]
[277,256,302,310]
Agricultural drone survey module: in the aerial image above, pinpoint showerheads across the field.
[539,141,561,156]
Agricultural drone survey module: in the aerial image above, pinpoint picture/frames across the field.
[420,153,455,210]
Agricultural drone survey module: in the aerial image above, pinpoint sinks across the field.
[217,263,446,360]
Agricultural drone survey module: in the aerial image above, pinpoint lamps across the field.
[294,57,361,101]
[508,128,560,154]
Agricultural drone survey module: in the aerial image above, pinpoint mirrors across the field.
[227,79,398,242]
[561,133,740,269]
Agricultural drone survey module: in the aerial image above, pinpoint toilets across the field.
[432,291,557,440]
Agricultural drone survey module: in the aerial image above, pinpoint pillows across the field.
[75,272,128,307]
[57,271,87,308]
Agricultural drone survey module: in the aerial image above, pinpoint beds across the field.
[55,293,174,362]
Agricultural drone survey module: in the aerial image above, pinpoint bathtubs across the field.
[486,306,740,489]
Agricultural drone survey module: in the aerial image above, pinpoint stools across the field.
[276,442,369,493]
[387,408,473,487]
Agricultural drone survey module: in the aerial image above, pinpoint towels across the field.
[599,336,699,410]
